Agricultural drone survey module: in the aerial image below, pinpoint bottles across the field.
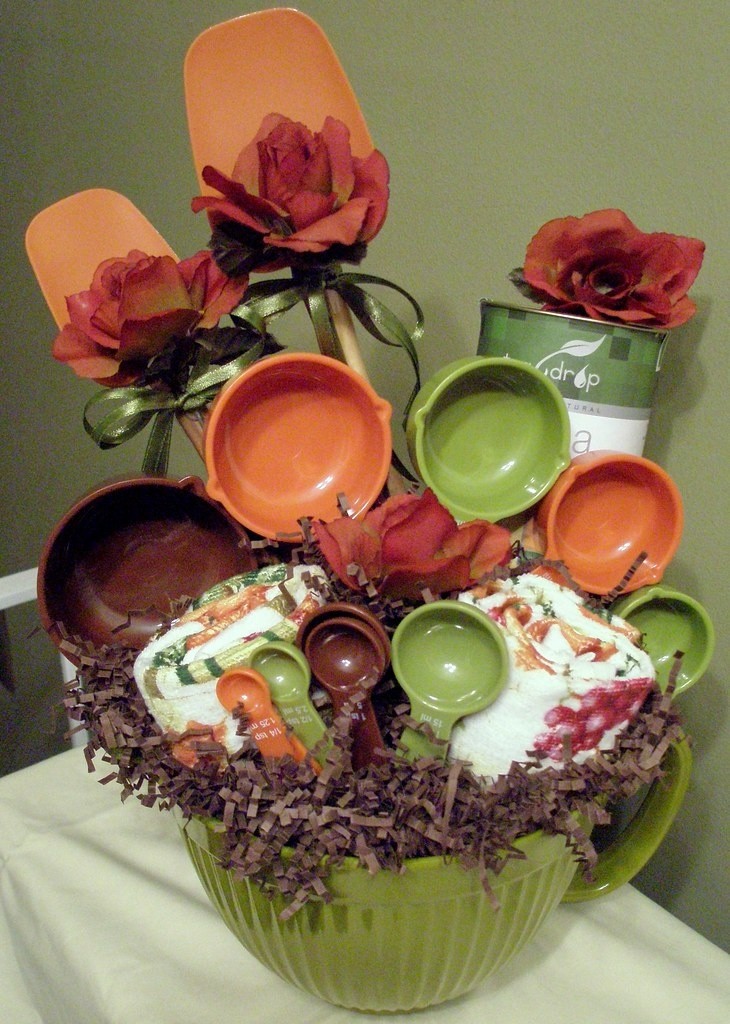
[469,287,678,571]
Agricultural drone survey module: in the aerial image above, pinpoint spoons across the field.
[215,667,315,776]
[249,642,336,770]
[388,597,508,771]
[303,618,384,736]
[290,603,391,773]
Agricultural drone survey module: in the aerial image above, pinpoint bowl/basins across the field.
[32,465,255,674]
[604,584,716,704]
[524,450,686,603]
[403,351,574,531]
[199,334,399,553]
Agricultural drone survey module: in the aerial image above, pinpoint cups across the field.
[160,714,695,1014]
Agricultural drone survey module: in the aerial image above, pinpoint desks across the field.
[0,732,728,1024]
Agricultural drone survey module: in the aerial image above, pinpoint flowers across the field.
[507,208,708,330]
[314,485,515,603]
[190,113,390,264]
[53,250,252,398]
